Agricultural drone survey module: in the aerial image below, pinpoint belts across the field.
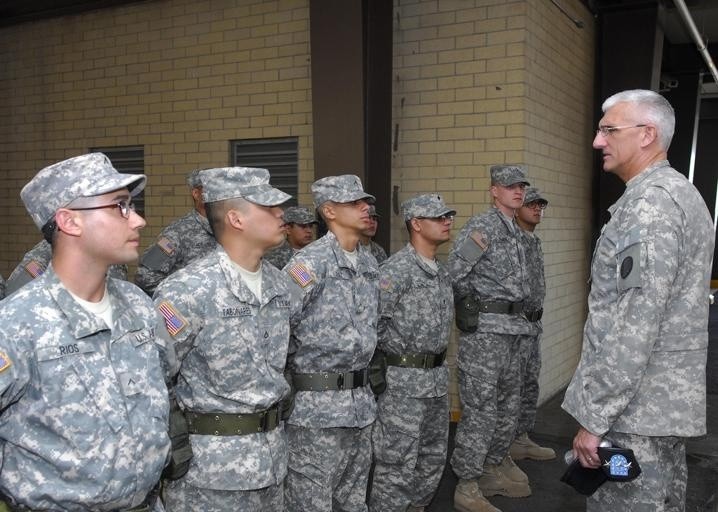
[294,368,367,391]
[184,402,282,437]
[479,300,524,314]
[528,308,543,321]
[388,349,447,368]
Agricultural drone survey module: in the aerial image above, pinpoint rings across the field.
[586,457,592,462]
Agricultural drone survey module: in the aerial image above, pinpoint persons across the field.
[445,162,533,512]
[4,233,130,303]
[558,87,715,511]
[369,190,460,512]
[1,151,175,510]
[360,201,390,270]
[262,205,320,272]
[497,184,558,483]
[279,173,381,512]
[131,167,225,297]
[152,164,294,510]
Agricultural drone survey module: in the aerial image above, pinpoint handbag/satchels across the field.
[370,356,386,393]
[456,295,477,332]
[167,397,194,480]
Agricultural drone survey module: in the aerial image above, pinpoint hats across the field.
[21,153,146,232]
[186,168,211,189]
[490,166,530,187]
[285,207,320,225]
[369,205,380,217]
[524,190,548,204]
[401,194,456,221]
[200,167,292,206]
[311,175,376,210]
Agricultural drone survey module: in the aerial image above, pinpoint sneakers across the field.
[511,433,556,461]
[482,465,531,497]
[502,455,529,482]
[454,483,502,512]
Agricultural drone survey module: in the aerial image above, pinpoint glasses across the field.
[418,215,454,222]
[524,201,547,209]
[596,125,645,135]
[71,201,136,219]
